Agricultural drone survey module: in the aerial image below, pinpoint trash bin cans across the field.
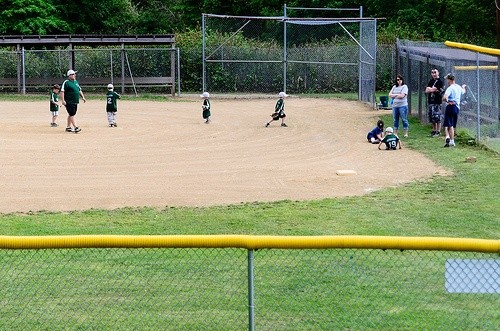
[380,94,389,108]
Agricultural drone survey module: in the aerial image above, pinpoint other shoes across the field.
[265,123,269,127]
[205,120,211,124]
[281,123,288,127]
[430,131,441,138]
[404,135,409,138]
[74,127,82,132]
[51,123,59,127]
[109,123,117,127]
[444,136,456,147]
[66,127,75,132]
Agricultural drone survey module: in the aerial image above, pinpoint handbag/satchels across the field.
[390,97,394,104]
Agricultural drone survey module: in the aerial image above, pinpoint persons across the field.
[266,92,288,127]
[367,120,385,144]
[442,73,466,147]
[61,70,86,133]
[200,92,211,123]
[425,68,443,137]
[106,84,120,127]
[388,75,409,138]
[378,127,401,150]
[50,84,61,126]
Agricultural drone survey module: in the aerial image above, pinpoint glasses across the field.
[397,78,402,81]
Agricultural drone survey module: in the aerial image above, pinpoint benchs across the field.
[0,78,174,95]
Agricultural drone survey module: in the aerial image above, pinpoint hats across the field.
[200,92,209,98]
[444,73,455,80]
[54,84,61,89]
[385,127,394,133]
[377,120,384,128]
[107,84,114,89]
[67,69,77,76]
[278,92,290,98]
